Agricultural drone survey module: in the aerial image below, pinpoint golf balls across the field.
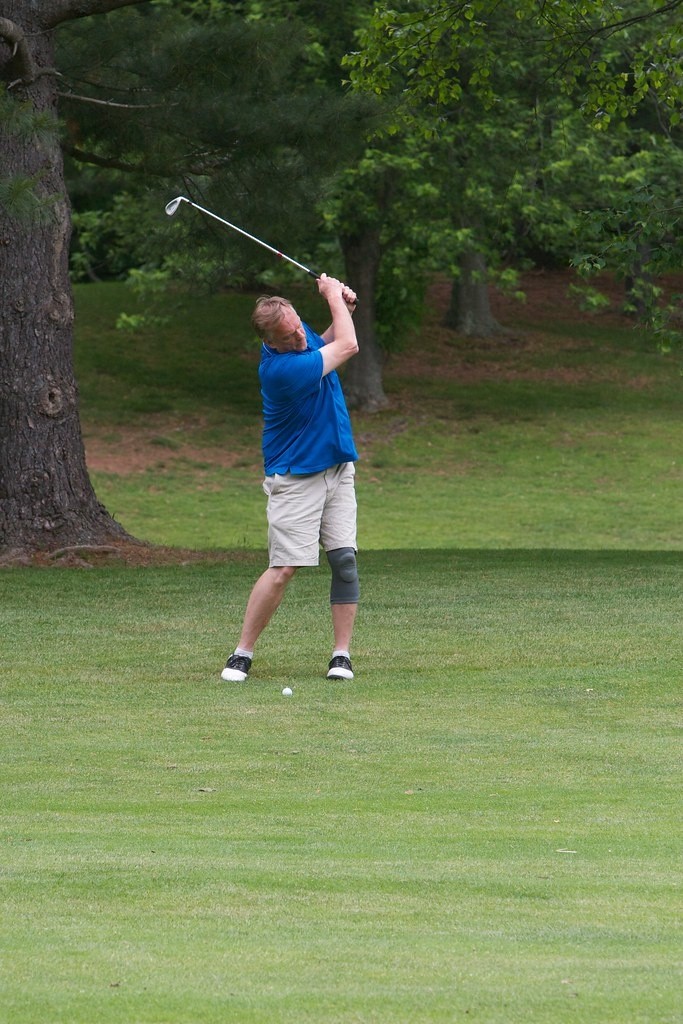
[282,687,293,696]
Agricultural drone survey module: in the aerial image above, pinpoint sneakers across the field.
[326,655,355,680]
[220,653,252,682]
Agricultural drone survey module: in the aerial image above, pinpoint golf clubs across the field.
[165,197,359,305]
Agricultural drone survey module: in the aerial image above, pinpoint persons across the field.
[220,272,359,684]
